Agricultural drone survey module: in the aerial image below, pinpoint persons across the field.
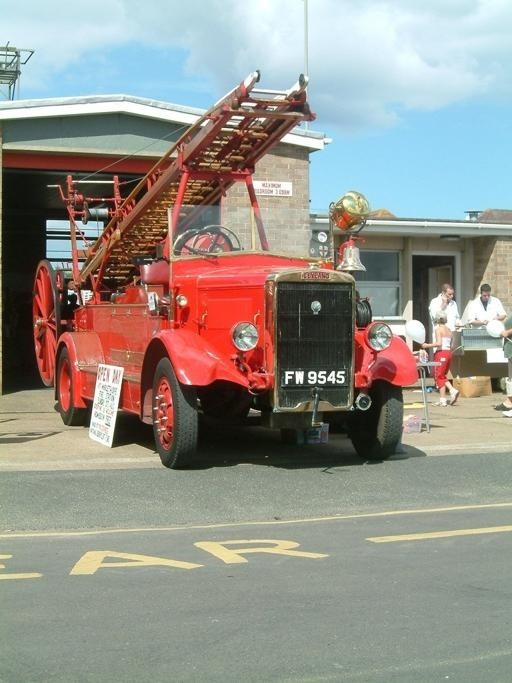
[422,311,461,408]
[492,309,512,411]
[461,284,507,327]
[428,283,460,330]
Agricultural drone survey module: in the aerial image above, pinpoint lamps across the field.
[440,235,460,241]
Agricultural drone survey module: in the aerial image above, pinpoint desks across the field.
[416,361,441,432]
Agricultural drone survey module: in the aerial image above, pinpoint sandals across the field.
[495,402,512,411]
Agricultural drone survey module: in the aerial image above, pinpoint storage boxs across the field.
[452,376,492,398]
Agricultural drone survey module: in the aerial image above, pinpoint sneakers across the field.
[449,389,459,405]
[431,399,449,408]
[501,411,512,417]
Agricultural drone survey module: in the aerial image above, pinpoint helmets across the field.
[435,311,447,323]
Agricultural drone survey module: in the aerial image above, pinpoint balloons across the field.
[405,316,427,345]
[487,319,505,339]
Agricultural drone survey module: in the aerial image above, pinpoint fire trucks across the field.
[31,70,419,469]
